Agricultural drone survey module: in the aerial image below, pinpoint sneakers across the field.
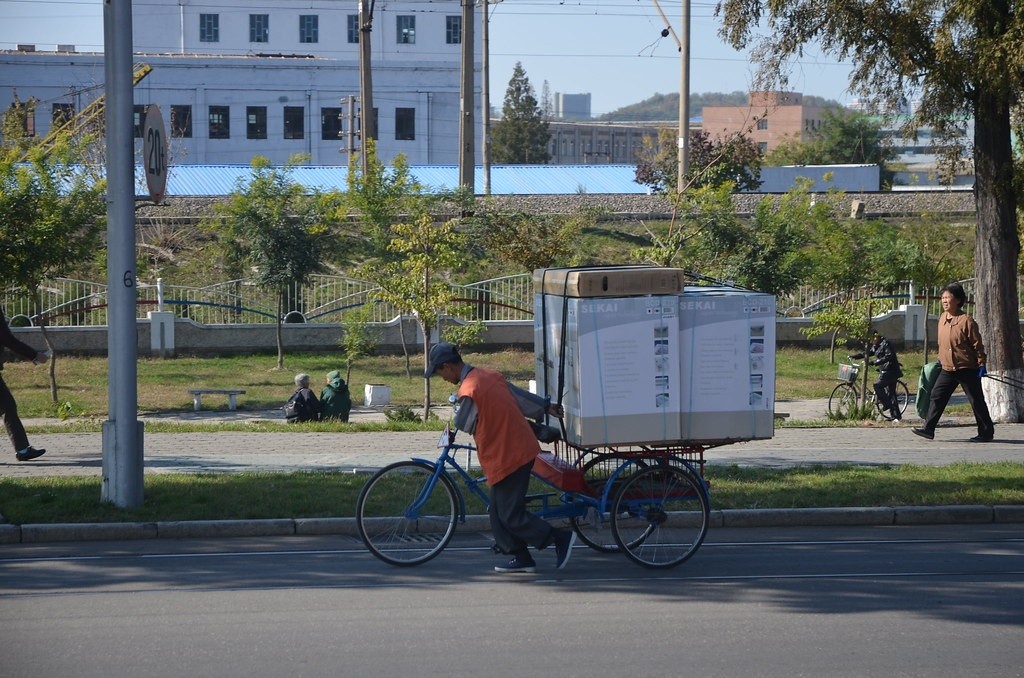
[494,556,536,573]
[555,530,577,570]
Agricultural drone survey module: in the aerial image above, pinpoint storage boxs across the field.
[674,280,777,442]
[532,269,686,450]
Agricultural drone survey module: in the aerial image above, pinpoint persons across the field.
[848,329,903,421]
[0,307,48,461]
[320,370,351,421]
[910,282,995,442]
[288,374,320,423]
[423,342,576,572]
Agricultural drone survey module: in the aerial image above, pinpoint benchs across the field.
[189,389,245,411]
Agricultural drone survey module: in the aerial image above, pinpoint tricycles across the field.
[355,394,713,572]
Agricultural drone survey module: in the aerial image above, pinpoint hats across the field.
[423,341,458,378]
[327,370,340,381]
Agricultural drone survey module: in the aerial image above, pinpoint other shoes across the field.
[882,403,892,411]
[16,446,45,461]
[970,435,993,443]
[885,417,901,422]
[911,427,934,439]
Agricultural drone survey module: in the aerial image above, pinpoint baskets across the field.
[837,363,857,383]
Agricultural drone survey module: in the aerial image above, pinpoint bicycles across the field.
[828,354,909,419]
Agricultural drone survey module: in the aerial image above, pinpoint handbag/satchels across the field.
[893,362,903,378]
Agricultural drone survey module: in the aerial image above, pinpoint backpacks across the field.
[283,388,306,423]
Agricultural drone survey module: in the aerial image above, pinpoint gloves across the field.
[850,355,857,359]
[938,360,941,365]
[977,366,987,379]
[869,361,874,366]
